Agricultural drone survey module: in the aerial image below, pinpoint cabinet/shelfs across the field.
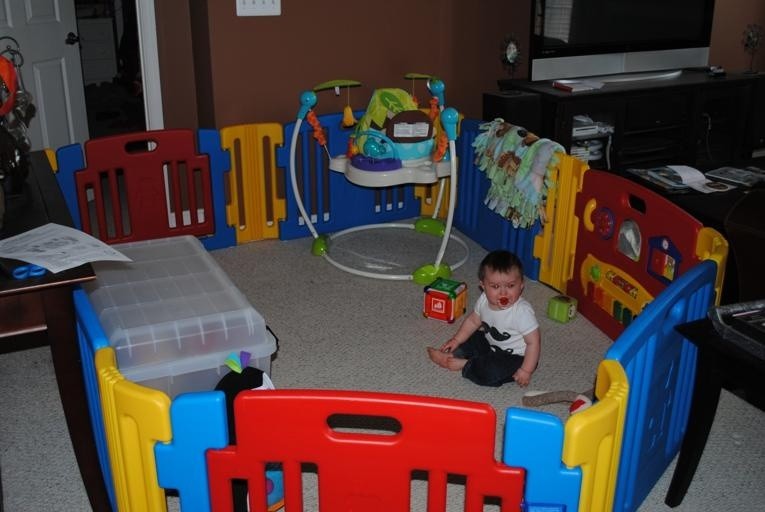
[508,67,765,171]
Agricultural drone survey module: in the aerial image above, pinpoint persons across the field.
[426,250,541,388]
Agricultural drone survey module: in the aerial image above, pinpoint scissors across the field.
[12,263,46,280]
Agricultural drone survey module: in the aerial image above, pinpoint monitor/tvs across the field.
[528,0,715,82]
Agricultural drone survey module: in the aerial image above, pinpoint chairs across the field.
[664,301,765,508]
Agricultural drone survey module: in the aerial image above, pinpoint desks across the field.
[0,150,114,512]
[626,164,765,202]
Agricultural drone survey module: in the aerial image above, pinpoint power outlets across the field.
[237,0,282,17]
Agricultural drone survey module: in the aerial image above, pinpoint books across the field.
[626,164,738,194]
[552,79,594,92]
